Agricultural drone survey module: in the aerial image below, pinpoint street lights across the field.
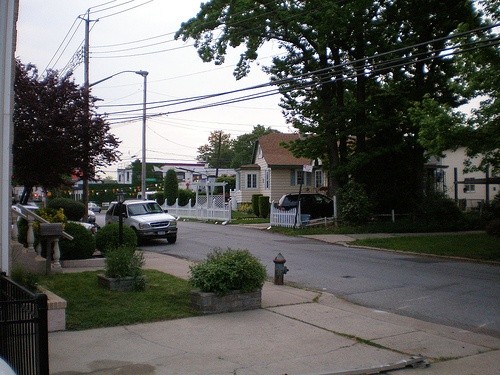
[81,70,150,221]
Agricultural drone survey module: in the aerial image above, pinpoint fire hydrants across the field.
[272,253,289,286]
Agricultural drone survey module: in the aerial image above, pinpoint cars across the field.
[88,203,102,213]
[80,210,97,223]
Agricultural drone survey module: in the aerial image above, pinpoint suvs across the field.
[276,192,336,220]
[104,199,178,247]
